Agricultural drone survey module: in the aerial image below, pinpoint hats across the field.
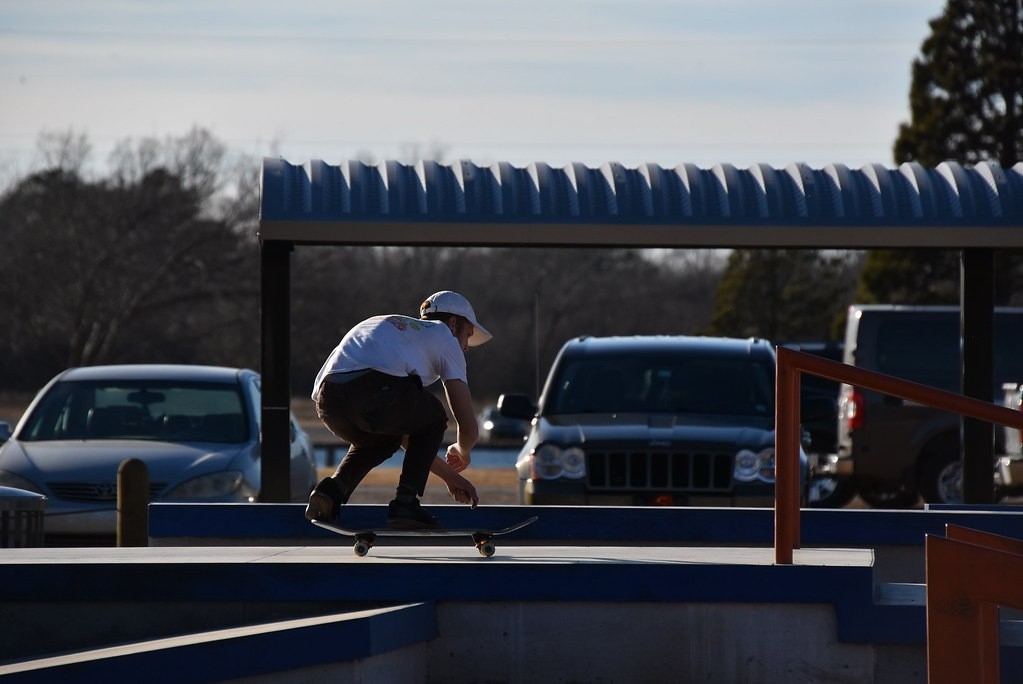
[420,289,493,346]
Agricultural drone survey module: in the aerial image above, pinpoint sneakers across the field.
[306,477,341,524]
[386,497,438,529]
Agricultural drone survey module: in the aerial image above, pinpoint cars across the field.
[477,407,528,446]
[0,362,318,550]
[497,334,823,509]
[780,341,847,451]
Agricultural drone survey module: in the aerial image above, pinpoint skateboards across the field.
[311,516,540,558]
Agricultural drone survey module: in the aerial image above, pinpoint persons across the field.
[305,290,496,531]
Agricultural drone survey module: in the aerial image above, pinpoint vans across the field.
[836,299,1023,508]
